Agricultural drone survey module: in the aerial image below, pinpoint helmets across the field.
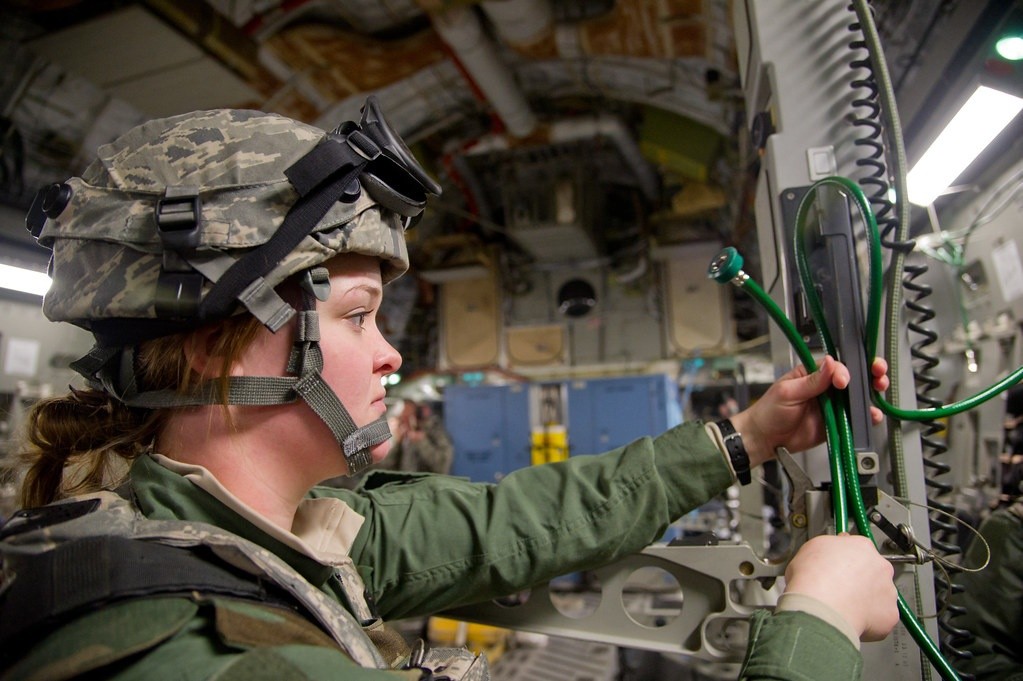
[26,108,408,321]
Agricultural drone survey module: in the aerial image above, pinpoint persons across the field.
[367,385,453,474]
[1,108,899,681]
[948,481,1023,681]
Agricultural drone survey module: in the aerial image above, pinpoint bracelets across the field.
[717,419,752,485]
